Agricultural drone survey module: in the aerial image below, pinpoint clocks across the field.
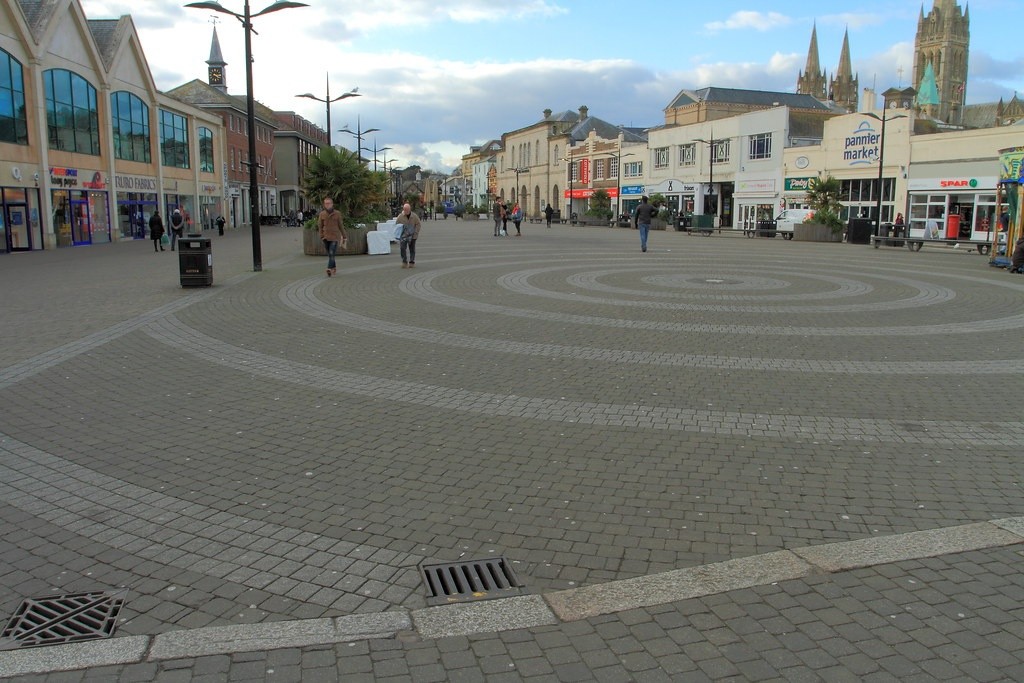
[890,100,898,108]
[903,101,909,108]
[209,67,222,84]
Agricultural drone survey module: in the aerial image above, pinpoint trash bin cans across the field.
[880,222,894,245]
[571,212,578,224]
[675,217,691,231]
[177,234,213,288]
[768,218,777,237]
[551,208,561,224]
[756,218,769,237]
[894,225,906,247]
[620,214,632,223]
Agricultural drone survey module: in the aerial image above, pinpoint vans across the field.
[773,209,820,239]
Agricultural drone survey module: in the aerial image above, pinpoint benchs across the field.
[529,217,620,227]
[873,236,1011,255]
[688,227,794,240]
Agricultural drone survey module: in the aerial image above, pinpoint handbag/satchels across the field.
[340,237,346,252]
[161,234,170,245]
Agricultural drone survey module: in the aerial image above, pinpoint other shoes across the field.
[332,267,336,273]
[327,269,331,275]
[402,262,407,268]
[515,233,521,236]
[155,250,158,252]
[161,249,165,251]
[409,263,413,268]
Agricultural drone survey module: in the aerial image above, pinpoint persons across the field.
[493,197,503,236]
[216,214,225,235]
[672,209,677,227]
[499,201,510,236]
[318,198,346,277]
[894,213,903,237]
[545,204,554,228]
[170,209,183,251]
[1000,206,1009,231]
[149,210,165,251]
[283,210,304,227]
[635,196,659,251]
[396,204,421,268]
[452,185,461,204]
[512,203,522,236]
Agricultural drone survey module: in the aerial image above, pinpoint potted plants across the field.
[435,200,517,221]
[793,178,848,242]
[303,153,391,256]
[577,187,613,227]
[631,194,672,231]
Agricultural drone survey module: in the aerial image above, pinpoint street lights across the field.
[859,112,908,244]
[293,92,363,145]
[602,152,635,228]
[359,146,392,172]
[507,167,518,203]
[185,0,310,271]
[337,129,380,165]
[691,138,732,214]
[371,158,404,211]
[563,158,587,224]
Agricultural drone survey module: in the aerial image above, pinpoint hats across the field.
[174,209,180,213]
[154,211,159,213]
